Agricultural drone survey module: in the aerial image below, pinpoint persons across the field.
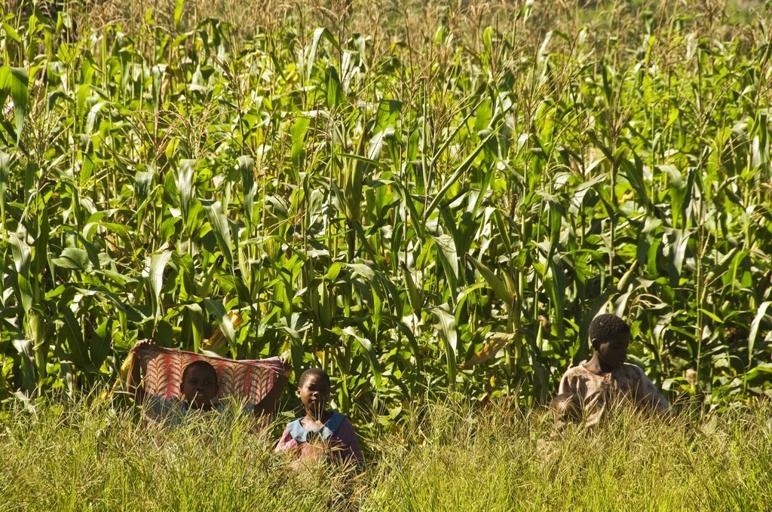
[275,368,363,503]
[552,392,583,430]
[551,313,674,450]
[126,339,294,442]
